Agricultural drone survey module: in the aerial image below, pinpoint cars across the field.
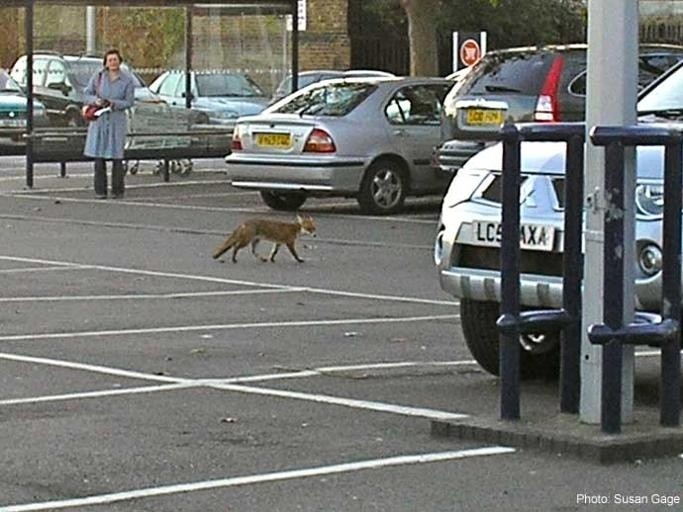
[223,74,460,214]
[0,65,51,150]
[270,67,398,103]
[137,70,273,138]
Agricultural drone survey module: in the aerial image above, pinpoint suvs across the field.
[433,58,683,380]
[433,42,683,180]
[6,50,174,126]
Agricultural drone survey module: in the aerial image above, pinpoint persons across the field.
[83,49,135,199]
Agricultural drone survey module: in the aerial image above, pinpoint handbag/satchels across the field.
[85,105,103,120]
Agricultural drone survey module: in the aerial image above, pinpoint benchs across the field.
[27,130,235,188]
